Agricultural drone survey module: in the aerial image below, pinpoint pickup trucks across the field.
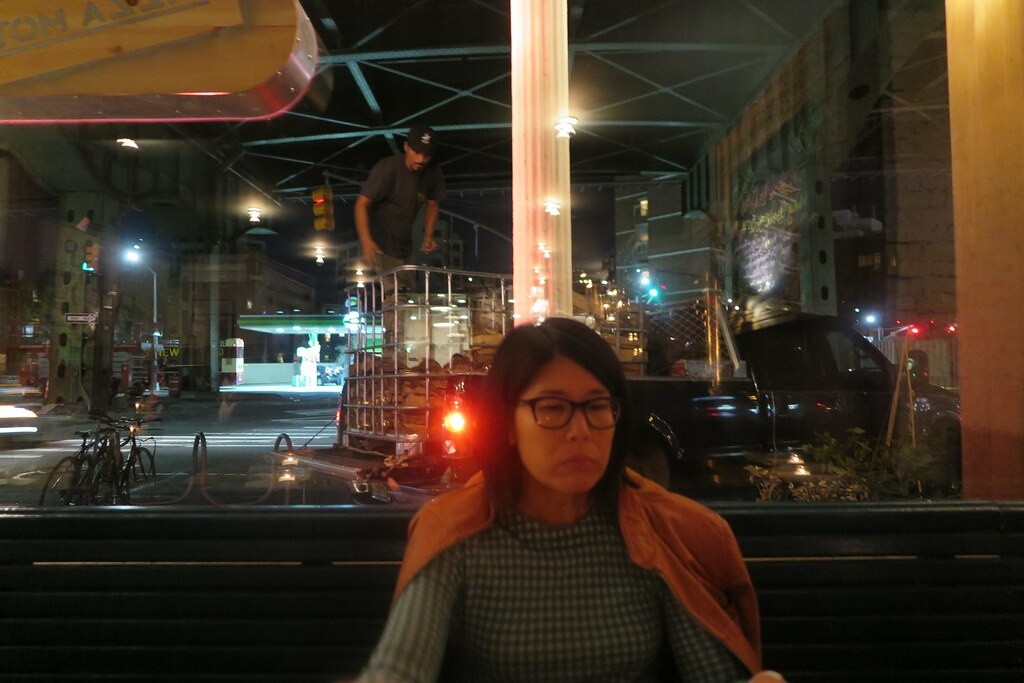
[275,296,962,507]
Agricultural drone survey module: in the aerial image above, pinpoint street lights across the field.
[124,249,160,390]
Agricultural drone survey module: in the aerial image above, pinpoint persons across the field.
[296,336,321,384]
[353,318,785,683]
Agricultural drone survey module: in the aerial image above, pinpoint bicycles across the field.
[38,405,164,507]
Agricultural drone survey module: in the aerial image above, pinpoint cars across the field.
[317,362,343,386]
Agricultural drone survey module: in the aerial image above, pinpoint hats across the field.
[407,125,437,156]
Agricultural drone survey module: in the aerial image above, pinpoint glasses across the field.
[519,396,622,430]
